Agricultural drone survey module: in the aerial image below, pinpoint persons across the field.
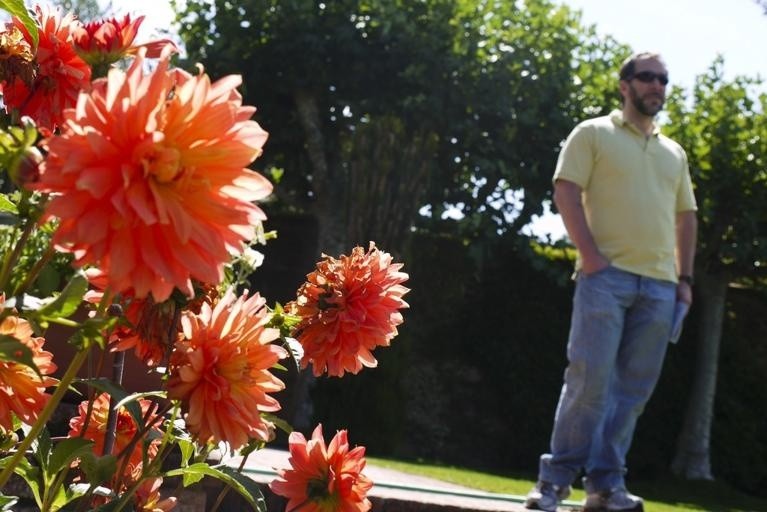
[525,53,700,512]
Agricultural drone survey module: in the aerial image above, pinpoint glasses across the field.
[626,71,668,85]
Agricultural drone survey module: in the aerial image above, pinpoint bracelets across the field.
[678,275,695,286]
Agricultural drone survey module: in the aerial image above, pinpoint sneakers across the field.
[584,485,643,512]
[523,479,570,512]
